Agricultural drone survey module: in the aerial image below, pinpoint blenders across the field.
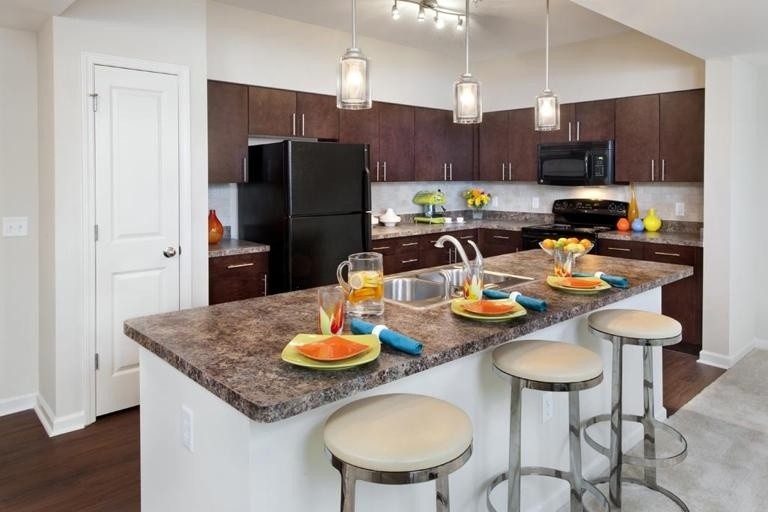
[413,191,445,224]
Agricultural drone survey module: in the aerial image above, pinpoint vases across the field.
[643,209,662,232]
[209,210,223,245]
[472,210,483,219]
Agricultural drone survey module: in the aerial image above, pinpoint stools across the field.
[485,339,616,512]
[586,308,689,512]
[323,395,471,512]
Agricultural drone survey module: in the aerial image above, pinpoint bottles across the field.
[628,192,639,222]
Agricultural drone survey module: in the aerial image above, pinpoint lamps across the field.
[337,2,372,109]
[534,1,563,131]
[388,0,472,31]
[454,0,485,125]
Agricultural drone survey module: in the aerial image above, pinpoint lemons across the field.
[564,244,584,252]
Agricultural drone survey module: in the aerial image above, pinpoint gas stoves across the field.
[521,222,609,234]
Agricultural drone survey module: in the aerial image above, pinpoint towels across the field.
[573,272,629,290]
[352,317,422,357]
[482,290,548,312]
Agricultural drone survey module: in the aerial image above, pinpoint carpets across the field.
[558,347,768,512]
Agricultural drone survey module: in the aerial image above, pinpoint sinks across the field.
[420,262,532,297]
[377,275,451,310]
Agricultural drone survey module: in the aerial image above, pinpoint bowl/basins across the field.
[538,241,594,260]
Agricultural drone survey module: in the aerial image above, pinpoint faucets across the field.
[433,235,484,299]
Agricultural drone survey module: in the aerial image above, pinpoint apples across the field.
[558,238,566,245]
[555,243,563,250]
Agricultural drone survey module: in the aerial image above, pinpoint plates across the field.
[547,274,612,295]
[461,300,515,315]
[294,336,369,361]
[559,278,602,288]
[280,333,381,371]
[450,298,528,323]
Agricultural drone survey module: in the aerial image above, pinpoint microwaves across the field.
[536,139,615,186]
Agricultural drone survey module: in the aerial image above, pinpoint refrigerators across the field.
[237,139,372,295]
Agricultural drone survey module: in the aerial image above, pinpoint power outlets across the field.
[1,216,28,238]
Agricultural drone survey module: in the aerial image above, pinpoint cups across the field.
[445,217,452,223]
[457,217,464,222]
[461,261,484,300]
[317,288,345,335]
[553,249,573,277]
[335,251,385,316]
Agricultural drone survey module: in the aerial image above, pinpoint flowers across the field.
[461,187,492,209]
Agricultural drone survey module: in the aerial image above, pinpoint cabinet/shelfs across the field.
[209,80,475,183]
[208,247,270,304]
[597,239,703,355]
[478,89,705,182]
[371,228,521,275]
